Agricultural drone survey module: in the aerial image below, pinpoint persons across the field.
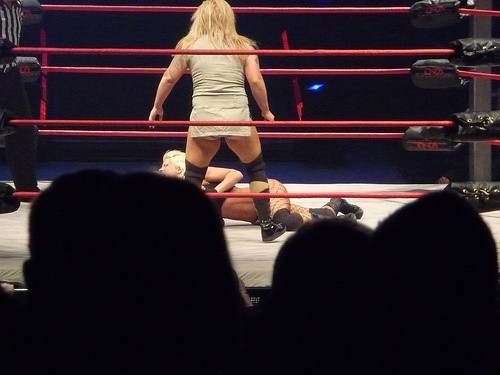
[149,0,286,242]
[0,169,500,375]
[159,150,363,231]
[0,0,41,202]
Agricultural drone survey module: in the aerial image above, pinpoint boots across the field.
[259,218,287,242]
[309,198,363,224]
